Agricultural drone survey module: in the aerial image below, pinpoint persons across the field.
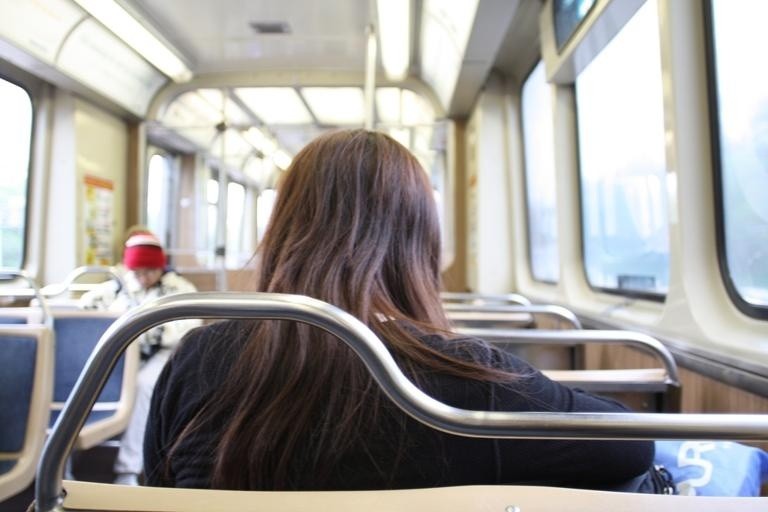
[142,130,655,492]
[77,228,203,484]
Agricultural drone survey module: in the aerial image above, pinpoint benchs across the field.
[1,265,768,512]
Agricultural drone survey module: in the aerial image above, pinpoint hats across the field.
[121,225,167,273]
